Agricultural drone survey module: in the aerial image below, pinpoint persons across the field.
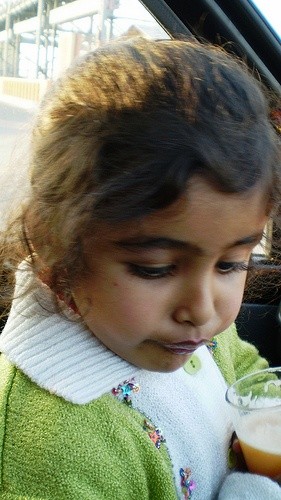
[1,35,281,500]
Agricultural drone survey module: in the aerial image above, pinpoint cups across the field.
[226,366,281,483]
